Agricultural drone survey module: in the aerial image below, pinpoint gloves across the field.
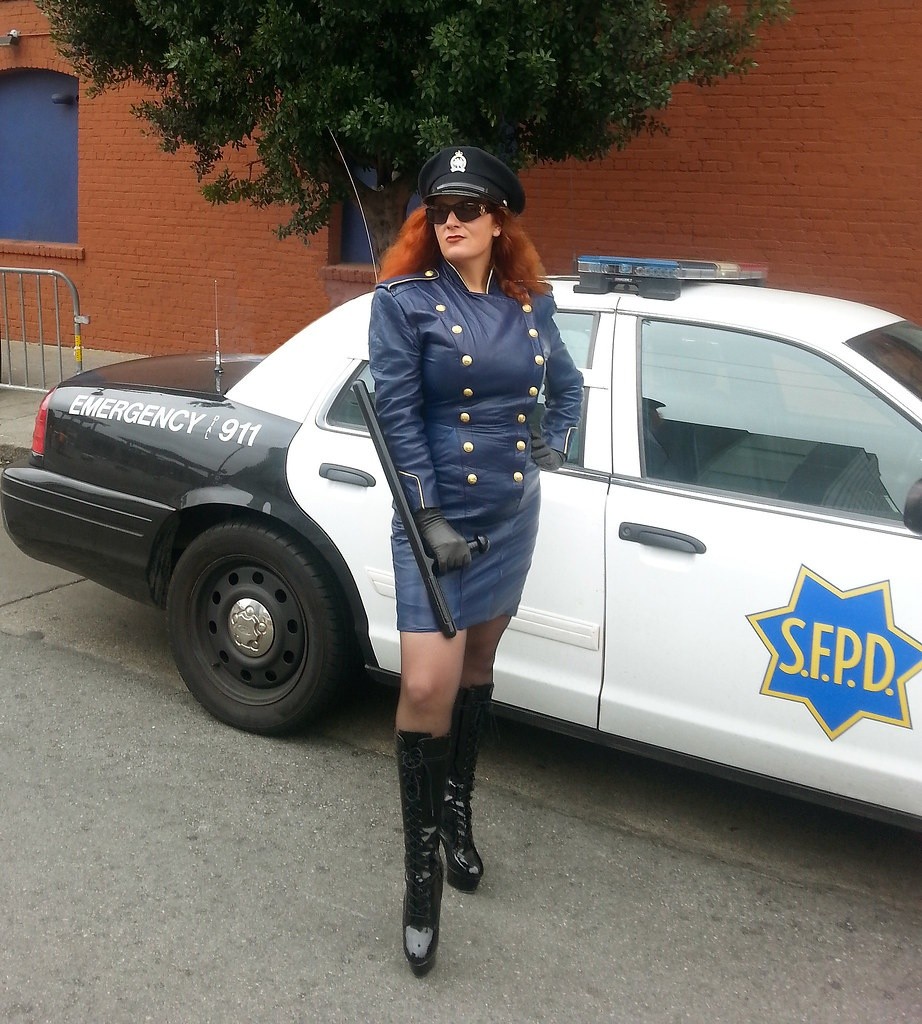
[412,507,473,575]
[531,430,566,471]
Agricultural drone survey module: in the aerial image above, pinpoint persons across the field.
[366,147,585,978]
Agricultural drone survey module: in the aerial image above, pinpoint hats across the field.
[418,146,525,218]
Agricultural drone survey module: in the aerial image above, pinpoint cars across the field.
[2,253,922,837]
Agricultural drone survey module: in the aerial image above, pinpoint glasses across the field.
[425,200,497,224]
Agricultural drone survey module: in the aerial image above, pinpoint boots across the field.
[438,682,494,891]
[393,730,447,978]
